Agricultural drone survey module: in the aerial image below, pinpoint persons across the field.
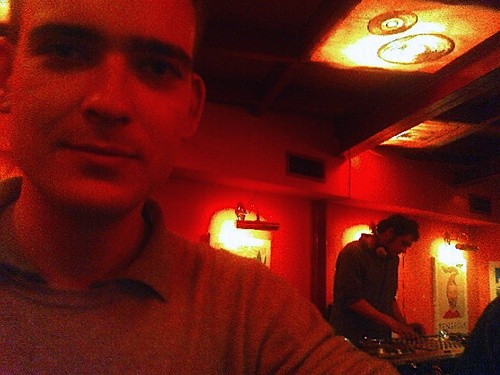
[455,297,500,375]
[0,0,401,375]
[333,214,427,356]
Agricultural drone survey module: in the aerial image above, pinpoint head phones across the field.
[372,227,388,258]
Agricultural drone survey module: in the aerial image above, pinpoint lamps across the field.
[454,231,481,252]
[235,207,281,231]
